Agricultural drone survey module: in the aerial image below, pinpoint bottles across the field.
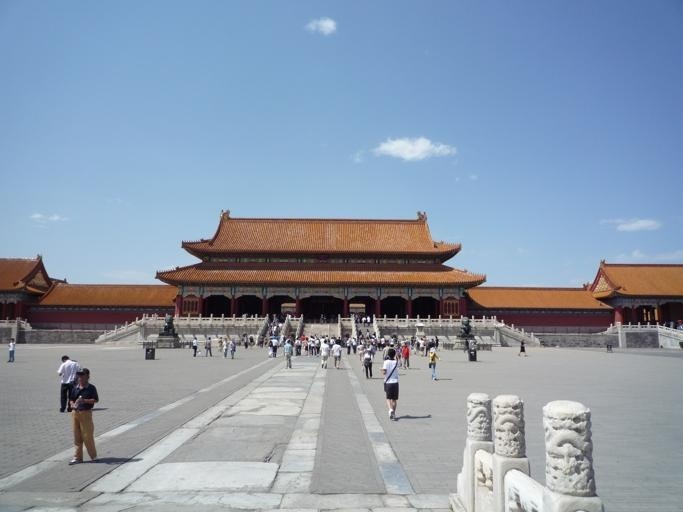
[73,396,82,408]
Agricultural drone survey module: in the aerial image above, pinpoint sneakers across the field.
[68,457,85,466]
[388,408,396,422]
[89,456,98,462]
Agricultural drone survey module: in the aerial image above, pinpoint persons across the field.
[7,338,16,362]
[380,349,400,421]
[68,368,99,465]
[192,312,469,380]
[518,339,528,357]
[57,355,81,412]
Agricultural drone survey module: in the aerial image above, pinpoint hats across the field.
[76,367,90,377]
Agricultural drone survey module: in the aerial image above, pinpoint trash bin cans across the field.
[468,349,476,361]
[606,345,612,351]
[145,347,154,360]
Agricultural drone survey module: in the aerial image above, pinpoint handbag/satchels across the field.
[428,362,432,368]
